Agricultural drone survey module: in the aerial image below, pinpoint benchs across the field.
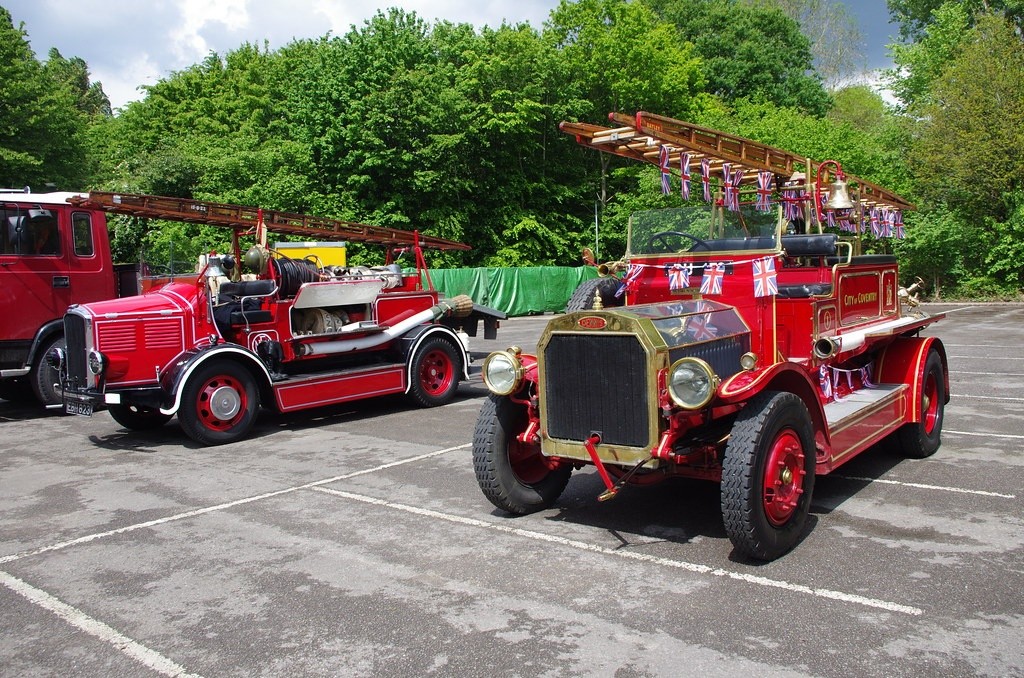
[210,280,278,325]
[682,234,854,302]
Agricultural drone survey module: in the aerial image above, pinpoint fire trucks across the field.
[470,109,952,563]
[46,188,507,448]
[0,185,211,407]
